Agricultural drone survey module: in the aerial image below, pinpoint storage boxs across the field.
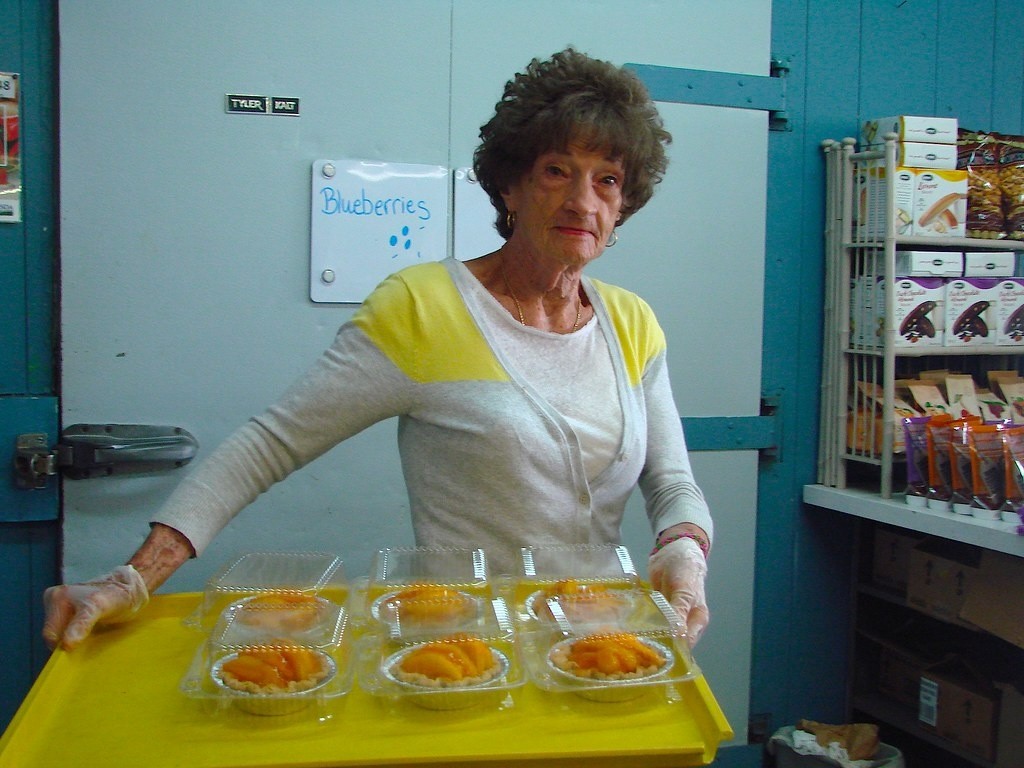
[851,114,1024,353]
[853,524,1024,762]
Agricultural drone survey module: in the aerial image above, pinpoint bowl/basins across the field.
[370,590,484,636]
[224,594,332,639]
[525,588,637,631]
[547,633,675,702]
[380,642,509,711]
[210,648,337,715]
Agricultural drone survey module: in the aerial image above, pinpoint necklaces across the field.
[500,263,582,334]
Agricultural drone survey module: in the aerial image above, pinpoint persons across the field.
[42,48,713,654]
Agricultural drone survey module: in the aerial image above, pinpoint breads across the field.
[795,718,880,762]
[899,194,1024,337]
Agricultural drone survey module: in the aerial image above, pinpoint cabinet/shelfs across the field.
[816,136,1024,500]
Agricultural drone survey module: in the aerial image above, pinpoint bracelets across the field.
[650,532,708,559]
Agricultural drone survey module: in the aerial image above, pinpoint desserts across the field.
[210,577,675,715]
